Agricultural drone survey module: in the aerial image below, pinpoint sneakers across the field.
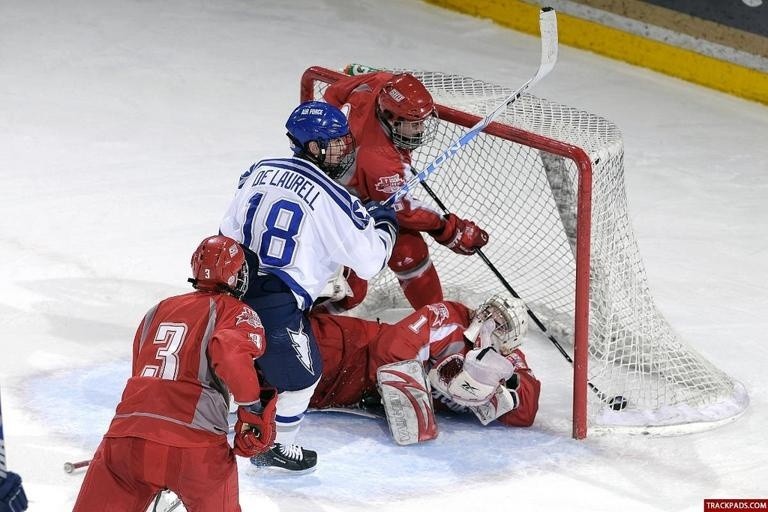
[251,443,317,471]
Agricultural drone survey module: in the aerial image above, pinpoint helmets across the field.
[377,72,434,121]
[469,295,527,355]
[285,100,352,146]
[190,235,245,297]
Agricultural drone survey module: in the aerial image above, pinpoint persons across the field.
[72,235,278,512]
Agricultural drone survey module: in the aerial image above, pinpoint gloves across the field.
[366,200,401,231]
[229,388,278,457]
[429,214,487,256]
